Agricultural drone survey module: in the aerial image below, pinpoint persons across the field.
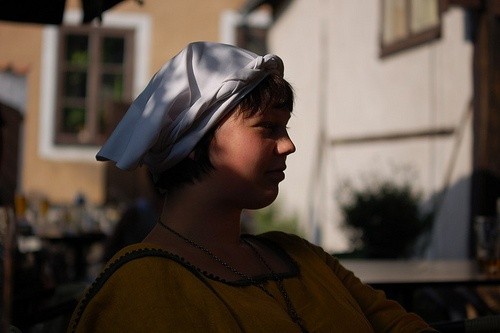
[72,39,447,333]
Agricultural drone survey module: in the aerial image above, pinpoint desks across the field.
[335,257,500,323]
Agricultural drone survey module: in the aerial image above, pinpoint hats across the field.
[95,42,284,183]
